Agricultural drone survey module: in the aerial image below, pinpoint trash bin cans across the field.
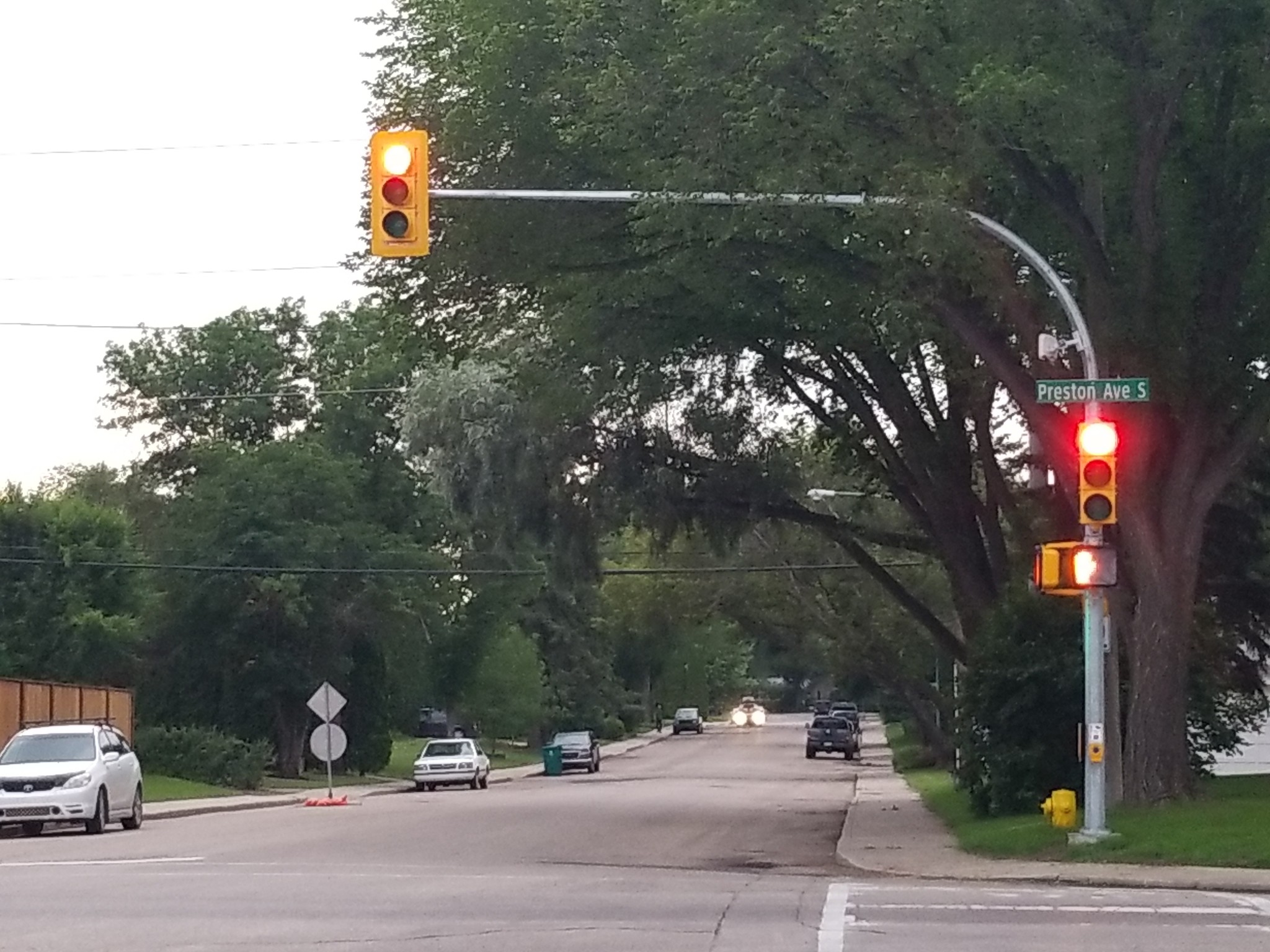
[543,744,564,775]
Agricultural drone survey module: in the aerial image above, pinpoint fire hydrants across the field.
[1040,788,1077,828]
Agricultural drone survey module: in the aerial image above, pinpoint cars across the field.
[552,729,601,774]
[731,703,766,727]
[672,707,703,735]
[804,715,856,761]
[0,716,143,840]
[412,738,491,792]
[812,698,860,734]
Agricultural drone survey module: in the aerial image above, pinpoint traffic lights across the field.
[369,130,430,258]
[1067,543,1118,588]
[1034,542,1060,591]
[1077,419,1118,525]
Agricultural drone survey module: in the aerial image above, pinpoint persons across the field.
[653,704,663,733]
